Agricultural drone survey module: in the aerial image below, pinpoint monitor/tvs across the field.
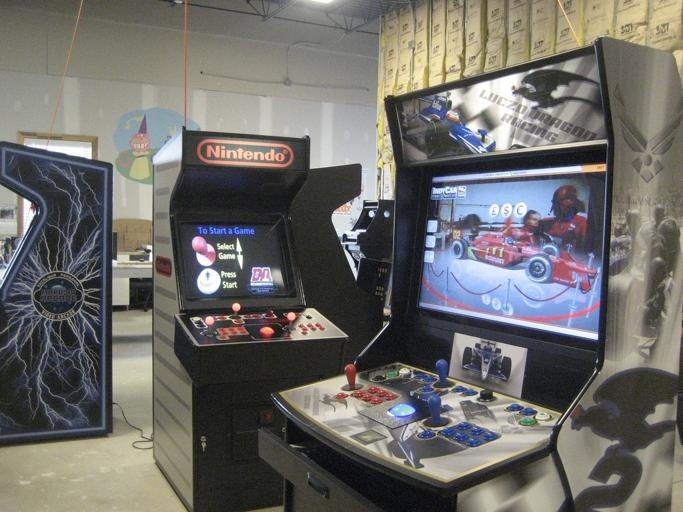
[176,220,293,298]
[407,149,608,351]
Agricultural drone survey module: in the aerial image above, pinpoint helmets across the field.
[551,184,579,219]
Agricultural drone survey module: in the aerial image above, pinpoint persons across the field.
[449,213,481,229]
[535,185,588,252]
[501,209,539,248]
